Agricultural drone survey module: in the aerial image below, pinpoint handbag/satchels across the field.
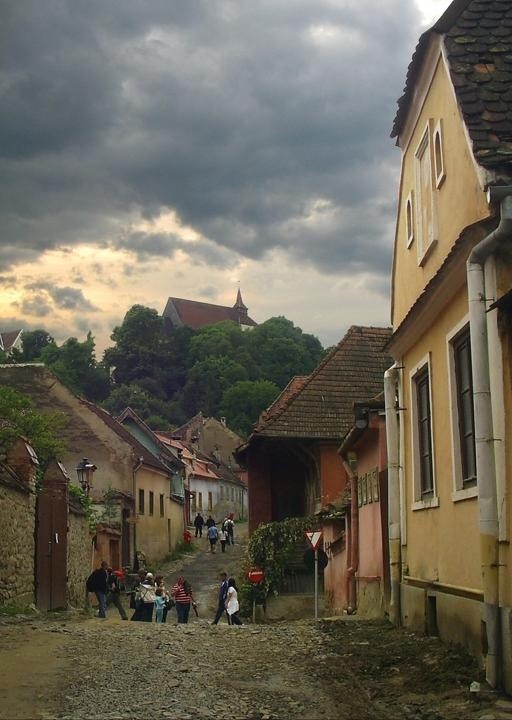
[227,520,233,532]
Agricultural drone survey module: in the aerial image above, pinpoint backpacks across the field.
[86,568,104,592]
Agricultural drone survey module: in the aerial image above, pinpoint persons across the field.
[171,576,197,623]
[95,566,129,621]
[130,572,155,621]
[221,518,228,534]
[154,588,167,623]
[129,566,147,621]
[206,515,215,528]
[206,522,219,554]
[154,575,171,624]
[210,572,229,626]
[223,516,236,546]
[217,526,229,552]
[193,512,205,538]
[93,560,109,618]
[223,577,243,625]
